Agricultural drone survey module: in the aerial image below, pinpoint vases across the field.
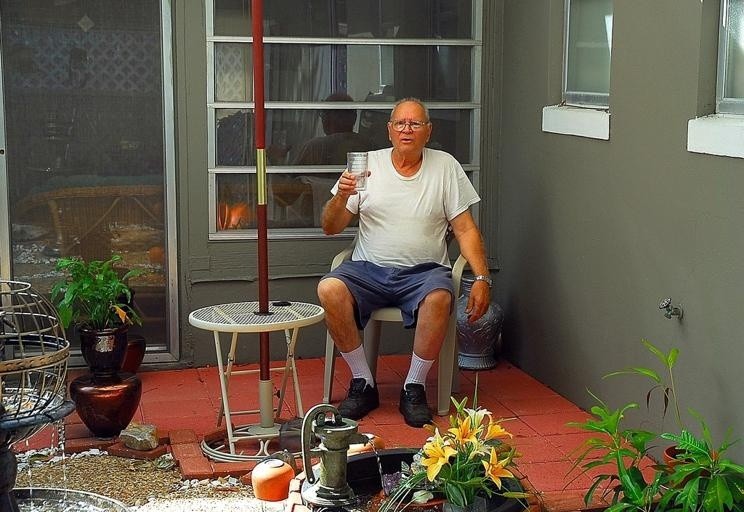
[454,274,504,371]
[443,495,486,512]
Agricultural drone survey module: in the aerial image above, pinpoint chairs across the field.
[11,184,166,331]
[218,181,314,229]
[323,228,486,417]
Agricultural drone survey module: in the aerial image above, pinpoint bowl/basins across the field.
[251,459,295,501]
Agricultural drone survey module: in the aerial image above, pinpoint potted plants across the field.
[602,337,718,489]
[51,254,148,380]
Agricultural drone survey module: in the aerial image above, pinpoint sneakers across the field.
[338,379,379,420]
[399,383,433,427]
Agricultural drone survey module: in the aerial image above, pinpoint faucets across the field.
[661,297,684,320]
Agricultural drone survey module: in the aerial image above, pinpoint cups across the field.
[347,152,368,191]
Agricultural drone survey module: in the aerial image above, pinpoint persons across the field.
[266,94,377,227]
[316,97,493,428]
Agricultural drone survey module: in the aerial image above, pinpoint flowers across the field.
[419,396,530,508]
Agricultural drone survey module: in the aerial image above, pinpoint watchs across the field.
[473,275,493,289]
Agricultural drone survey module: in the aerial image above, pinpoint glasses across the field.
[390,121,430,132]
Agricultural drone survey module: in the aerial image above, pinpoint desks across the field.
[189,300,325,464]
